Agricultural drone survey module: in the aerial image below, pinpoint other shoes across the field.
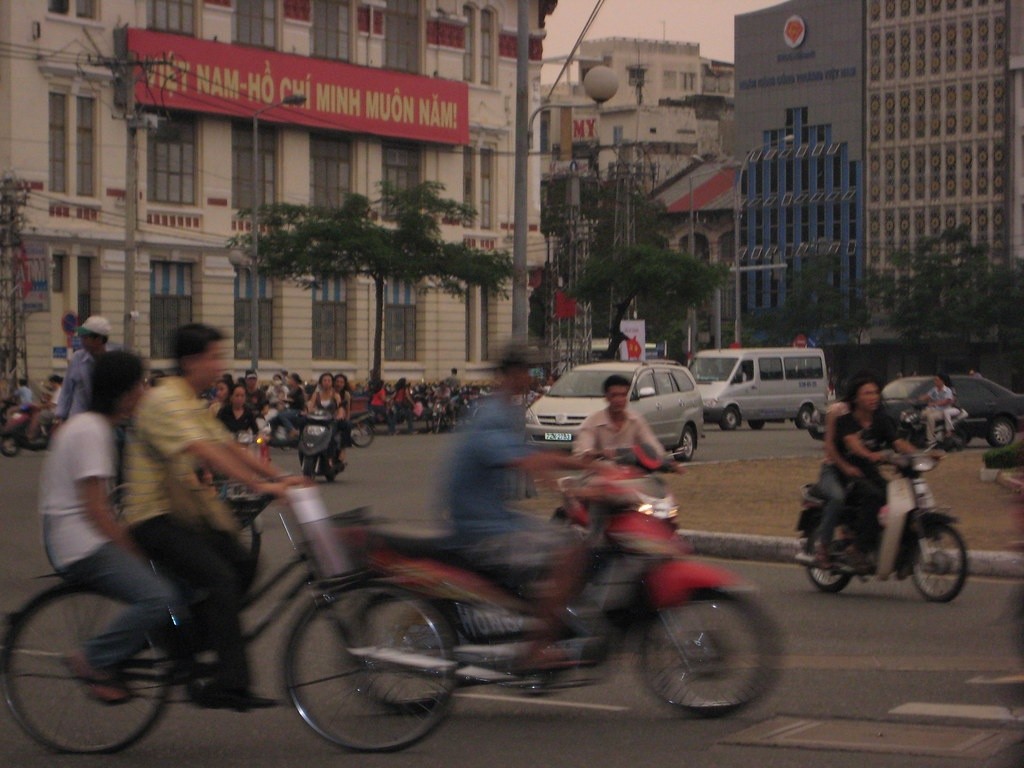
[814,550,833,569]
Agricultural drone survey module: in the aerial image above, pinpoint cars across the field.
[806,375,1024,447]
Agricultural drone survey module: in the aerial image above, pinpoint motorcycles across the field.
[350,410,376,449]
[550,443,693,560]
[792,437,969,602]
[332,455,785,717]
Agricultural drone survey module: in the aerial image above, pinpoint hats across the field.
[77,315,111,338]
[245,369,259,381]
[497,344,550,370]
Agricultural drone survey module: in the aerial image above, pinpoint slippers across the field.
[63,656,132,707]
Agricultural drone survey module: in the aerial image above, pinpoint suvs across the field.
[524,360,705,462]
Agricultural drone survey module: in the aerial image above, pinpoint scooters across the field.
[0,404,62,458]
[216,429,272,525]
[297,409,351,482]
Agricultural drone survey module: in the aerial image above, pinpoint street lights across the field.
[251,94,307,371]
[511,66,621,354]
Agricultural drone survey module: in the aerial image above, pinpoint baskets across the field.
[295,504,376,590]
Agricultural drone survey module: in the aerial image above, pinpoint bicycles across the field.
[1,478,456,757]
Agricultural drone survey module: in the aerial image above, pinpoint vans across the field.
[688,347,828,430]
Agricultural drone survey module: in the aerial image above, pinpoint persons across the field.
[6,375,63,441]
[121,315,311,709]
[435,341,611,669]
[810,372,943,571]
[38,348,190,705]
[197,368,460,470]
[573,374,673,551]
[969,367,982,378]
[54,316,110,422]
[909,373,960,448]
